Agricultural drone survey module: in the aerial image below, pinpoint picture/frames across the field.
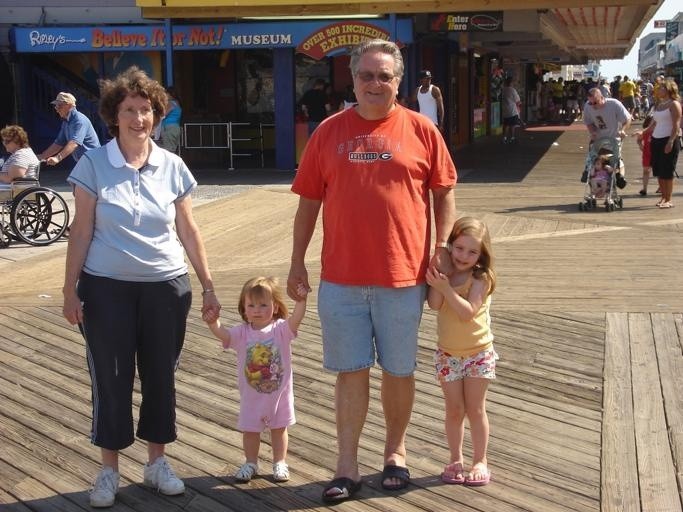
[234,48,334,129]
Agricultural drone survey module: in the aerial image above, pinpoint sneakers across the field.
[655,200,674,209]
[639,190,647,195]
[142,455,186,497]
[88,466,120,509]
[234,462,259,481]
[502,136,516,143]
[271,460,290,482]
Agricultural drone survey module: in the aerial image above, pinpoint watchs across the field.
[435,242,452,254]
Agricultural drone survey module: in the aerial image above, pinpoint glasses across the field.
[357,71,397,83]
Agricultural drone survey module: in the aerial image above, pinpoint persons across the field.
[301,78,357,138]
[501,78,521,145]
[60,65,222,510]
[411,69,445,134]
[36,91,102,238]
[425,216,498,486]
[285,37,459,504]
[159,86,183,154]
[540,75,683,209]
[201,275,309,483]
[0,123,39,185]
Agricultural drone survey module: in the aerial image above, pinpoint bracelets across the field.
[58,153,63,162]
[202,289,214,294]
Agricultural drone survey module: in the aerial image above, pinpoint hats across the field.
[417,69,435,80]
[49,92,77,105]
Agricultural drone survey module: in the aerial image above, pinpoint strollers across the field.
[578,133,629,214]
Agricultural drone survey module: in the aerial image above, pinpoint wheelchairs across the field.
[1,158,72,250]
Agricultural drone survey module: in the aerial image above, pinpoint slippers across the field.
[381,464,411,490]
[464,468,492,486]
[442,462,465,485]
[321,478,364,502]
